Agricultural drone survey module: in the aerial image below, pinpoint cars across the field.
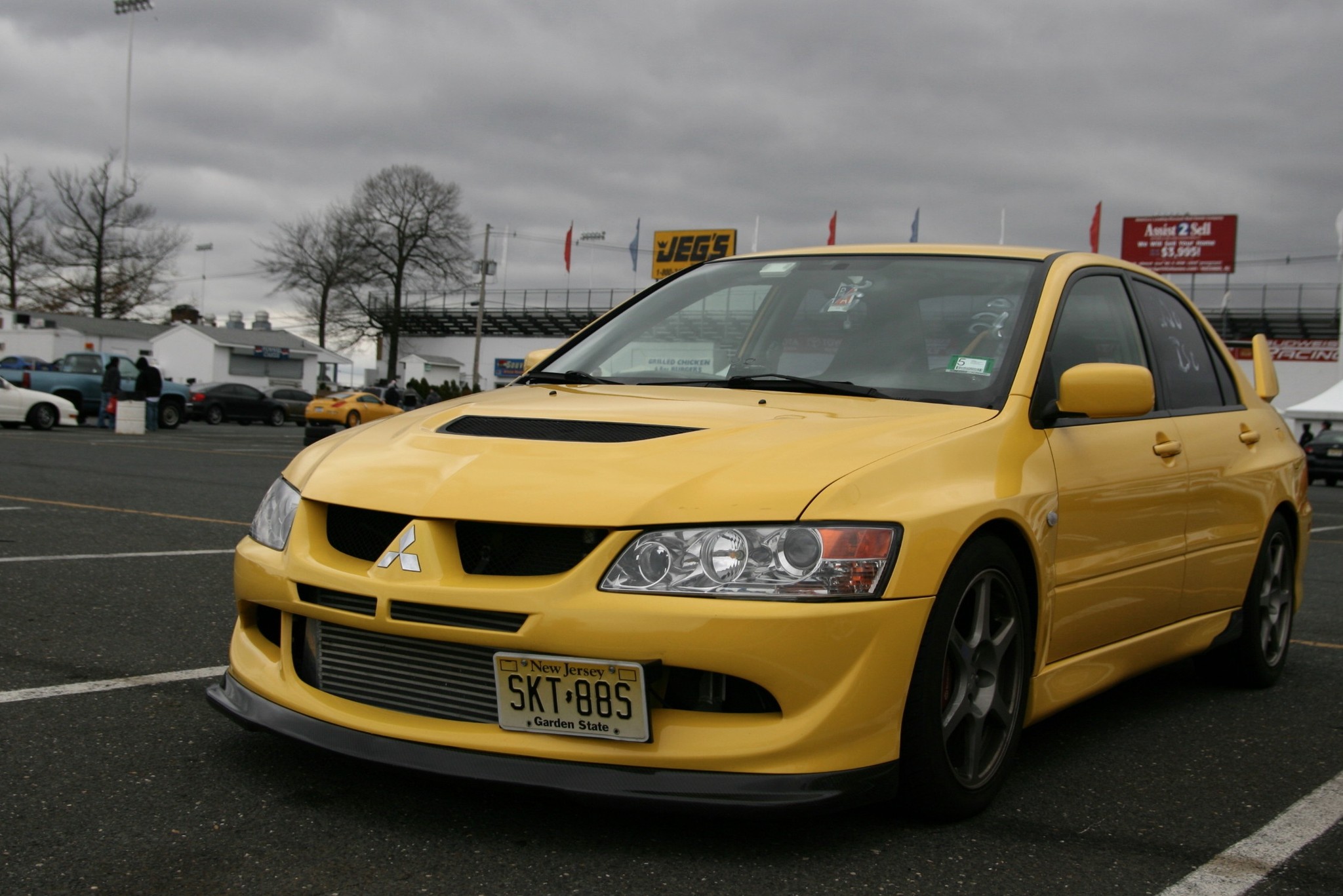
[0,376,79,431]
[1302,427,1343,487]
[204,245,1315,829]
[189,383,290,427]
[263,386,315,427]
[0,355,59,372]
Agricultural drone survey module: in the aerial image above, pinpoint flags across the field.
[565,226,573,272]
[502,226,508,270]
[828,215,835,245]
[630,220,639,271]
[1089,203,1101,254]
[1000,212,1005,244]
[751,216,758,252]
[910,209,919,242]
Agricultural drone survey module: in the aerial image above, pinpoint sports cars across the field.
[304,391,406,429]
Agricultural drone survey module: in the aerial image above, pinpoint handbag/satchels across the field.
[106,396,118,414]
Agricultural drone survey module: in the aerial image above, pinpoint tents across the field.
[1285,380,1343,440]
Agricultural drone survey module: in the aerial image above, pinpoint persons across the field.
[1298,424,1313,447]
[426,387,442,405]
[392,380,397,388]
[98,357,120,428]
[134,357,162,430]
[1319,419,1331,435]
[382,383,400,406]
[402,383,418,411]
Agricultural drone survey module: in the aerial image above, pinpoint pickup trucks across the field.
[0,349,191,430]
